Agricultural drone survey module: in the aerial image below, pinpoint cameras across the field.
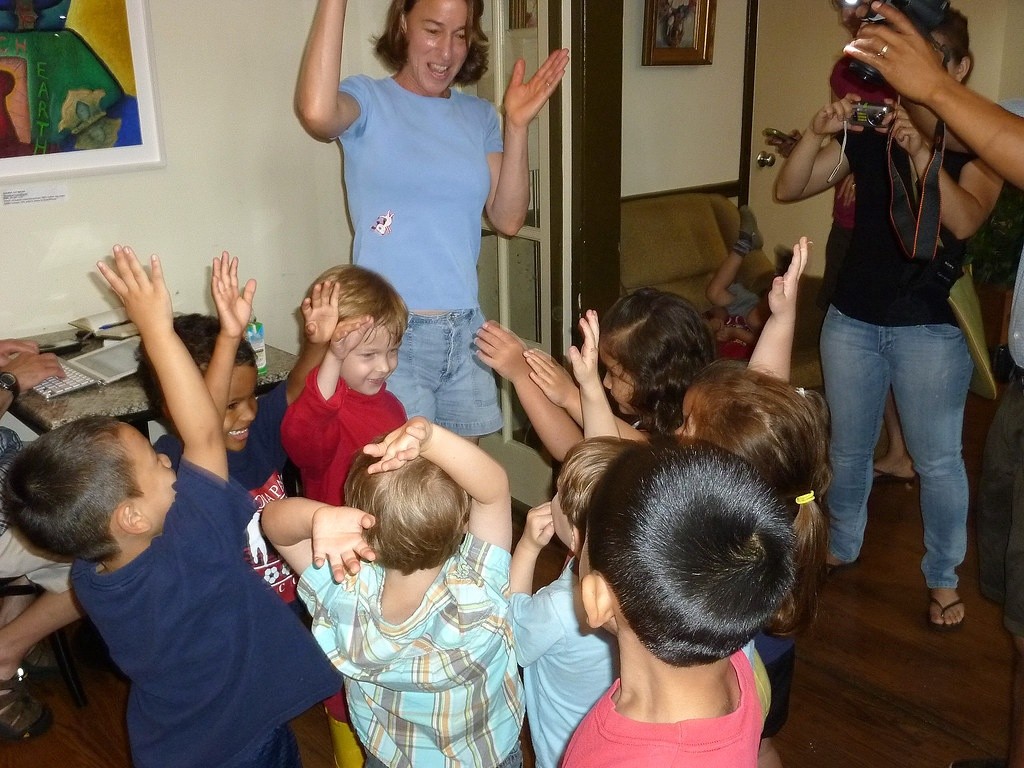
[849,102,891,128]
[850,0,951,84]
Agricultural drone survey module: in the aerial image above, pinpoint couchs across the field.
[619,192,828,395]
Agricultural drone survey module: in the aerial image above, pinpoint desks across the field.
[3,322,298,439]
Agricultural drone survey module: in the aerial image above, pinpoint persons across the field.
[138,248,342,633]
[280,264,408,505]
[843,0,1024,768]
[261,417,526,768]
[0,340,82,741]
[293,0,569,448]
[706,206,798,362]
[776,1,1003,632]
[0,245,343,768]
[561,437,799,768]
[477,237,828,768]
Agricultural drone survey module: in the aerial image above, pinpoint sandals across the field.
[0,672,54,741]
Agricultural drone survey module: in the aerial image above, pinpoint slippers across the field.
[822,557,860,582]
[872,461,915,484]
[926,595,966,632]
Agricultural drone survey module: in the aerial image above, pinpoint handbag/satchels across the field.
[950,265,999,400]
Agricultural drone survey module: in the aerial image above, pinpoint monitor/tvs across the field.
[68,334,146,384]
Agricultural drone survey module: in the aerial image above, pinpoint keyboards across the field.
[30,352,106,399]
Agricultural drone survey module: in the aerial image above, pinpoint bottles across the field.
[241,314,267,374]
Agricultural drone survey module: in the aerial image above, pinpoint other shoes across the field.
[738,206,764,248]
[774,244,795,274]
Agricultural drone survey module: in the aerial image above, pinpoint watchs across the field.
[0,372,20,399]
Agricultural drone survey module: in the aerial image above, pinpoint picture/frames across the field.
[641,0,717,66]
[0,0,167,182]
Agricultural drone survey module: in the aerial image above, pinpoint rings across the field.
[852,183,856,187]
[545,81,550,86]
[880,43,890,57]
